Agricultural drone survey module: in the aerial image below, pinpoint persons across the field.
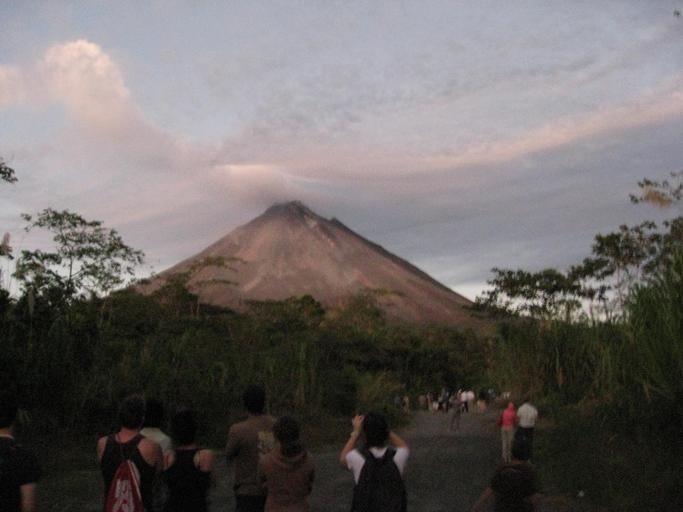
[1,402,36,512]
[401,388,538,464]
[227,384,317,512]
[339,412,410,511]
[469,442,538,512]
[97,396,214,512]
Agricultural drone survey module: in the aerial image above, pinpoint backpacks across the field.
[104,434,146,512]
[351,446,405,511]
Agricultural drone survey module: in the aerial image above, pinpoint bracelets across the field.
[351,431,360,436]
